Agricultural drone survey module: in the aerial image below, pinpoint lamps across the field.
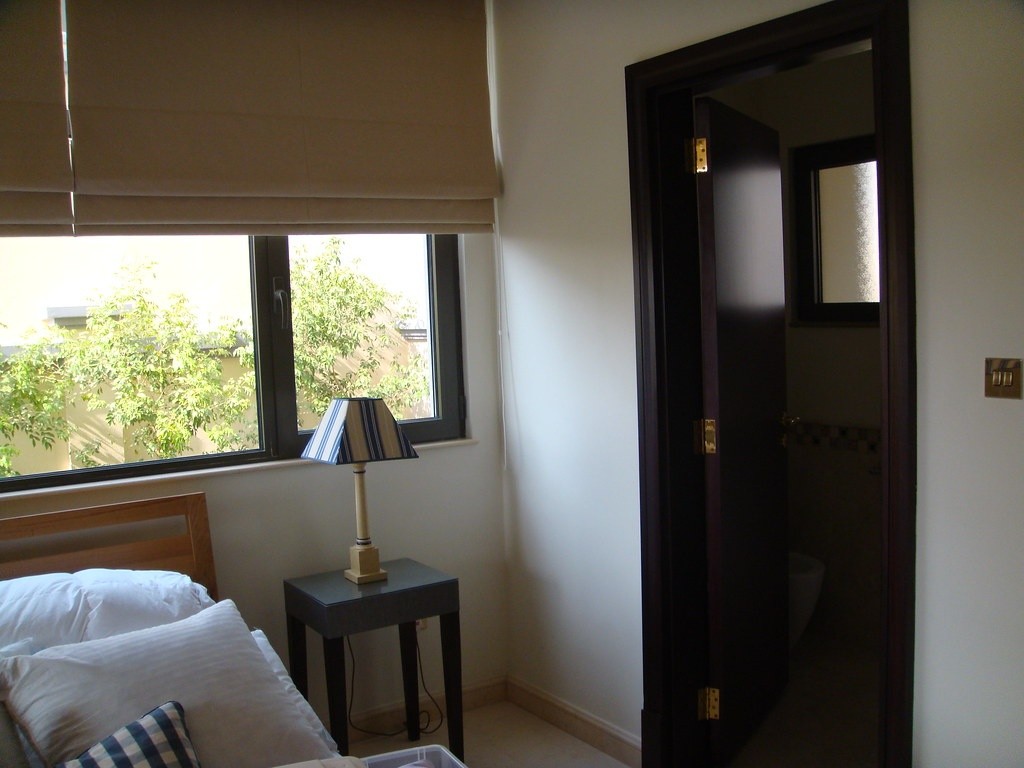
[302,395,420,581]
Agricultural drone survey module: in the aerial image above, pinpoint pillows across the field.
[62,700,200,768]
[0,597,333,768]
[250,627,339,749]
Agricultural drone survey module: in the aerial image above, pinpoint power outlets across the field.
[416,617,428,631]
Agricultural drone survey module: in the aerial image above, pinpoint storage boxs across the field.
[361,744,469,768]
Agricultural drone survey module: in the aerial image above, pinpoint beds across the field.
[0,487,361,768]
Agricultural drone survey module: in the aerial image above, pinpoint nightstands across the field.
[282,558,464,755]
